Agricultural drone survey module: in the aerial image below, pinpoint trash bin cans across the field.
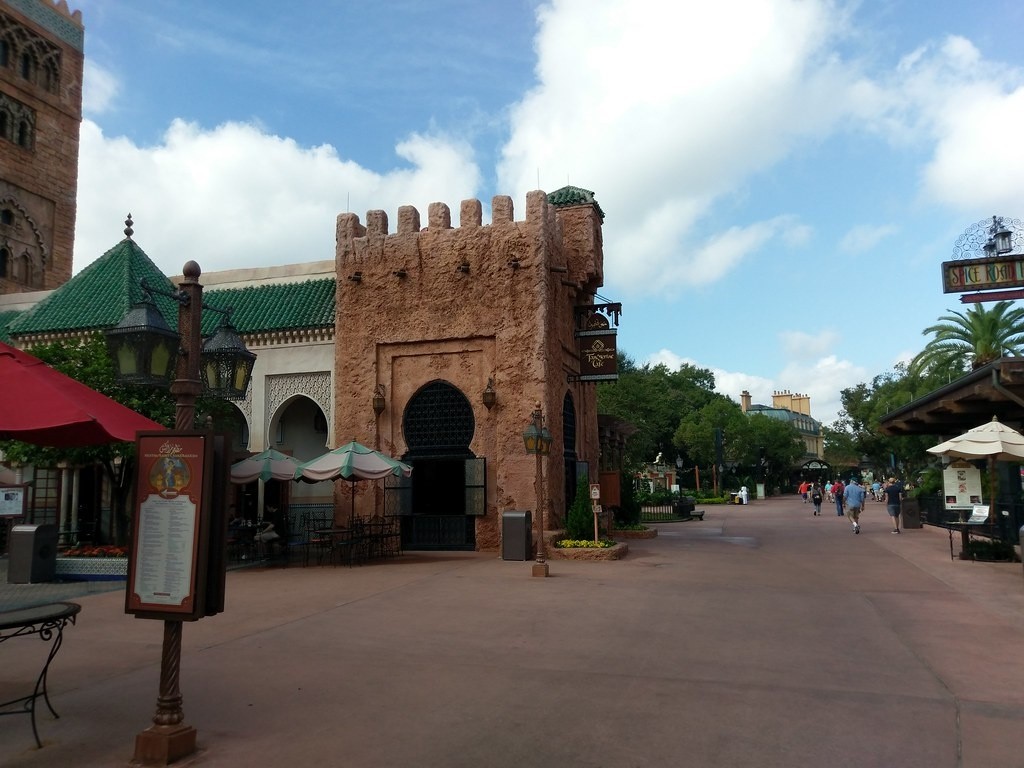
[502,510,532,561]
[7,523,59,583]
[899,497,921,529]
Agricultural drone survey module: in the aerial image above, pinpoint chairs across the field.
[259,511,403,568]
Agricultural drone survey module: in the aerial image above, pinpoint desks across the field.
[945,521,996,561]
[1,602,81,747]
[353,532,400,560]
[316,529,354,564]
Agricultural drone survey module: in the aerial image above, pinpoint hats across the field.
[849,477,858,482]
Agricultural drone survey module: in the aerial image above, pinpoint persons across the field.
[741,484,747,505]
[228,504,241,532]
[873,479,882,502]
[884,477,903,534]
[862,482,866,498]
[843,477,865,534]
[798,481,812,503]
[825,480,833,501]
[254,499,284,561]
[811,482,824,516]
[831,478,844,517]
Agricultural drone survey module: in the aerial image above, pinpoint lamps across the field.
[482,376,496,411]
[373,384,386,416]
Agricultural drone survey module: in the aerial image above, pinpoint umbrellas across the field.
[294,438,414,523]
[926,414,1024,525]
[230,448,305,484]
[0,341,166,445]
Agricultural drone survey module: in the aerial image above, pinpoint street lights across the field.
[107,258,258,767]
[523,401,552,577]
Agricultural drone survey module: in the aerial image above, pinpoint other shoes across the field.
[891,529,899,535]
[853,525,860,535]
[818,513,820,515]
[814,511,816,516]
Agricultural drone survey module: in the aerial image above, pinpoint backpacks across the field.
[835,486,842,498]
